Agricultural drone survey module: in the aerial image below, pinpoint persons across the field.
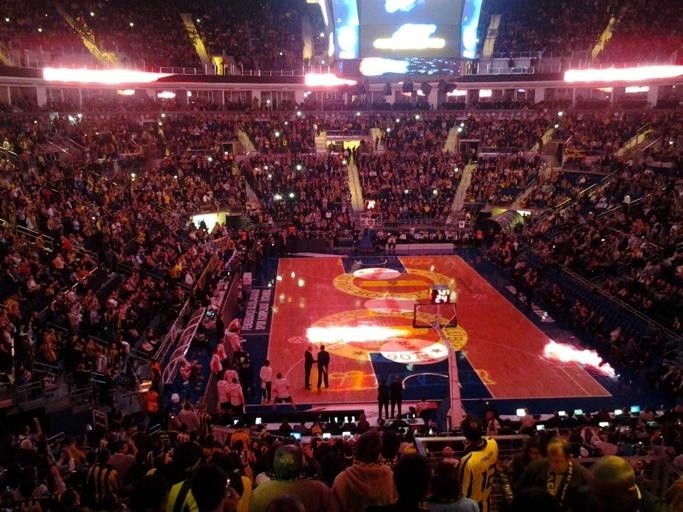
[376,372,389,422]
[304,347,315,389]
[482,237,683,392]
[217,407,683,512]
[226,0,683,249]
[317,345,329,388]
[390,375,403,417]
[223,317,251,368]
[216,368,243,404]
[230,244,268,266]
[271,370,290,407]
[259,360,273,399]
[2,1,232,512]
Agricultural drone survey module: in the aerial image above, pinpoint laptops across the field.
[630,405,640,416]
[289,432,301,441]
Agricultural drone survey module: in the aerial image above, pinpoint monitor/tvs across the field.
[322,433,331,440]
[558,410,566,417]
[342,431,350,436]
[255,417,262,425]
[536,424,545,430]
[614,409,624,417]
[598,421,609,428]
[516,408,525,416]
[574,409,583,416]
